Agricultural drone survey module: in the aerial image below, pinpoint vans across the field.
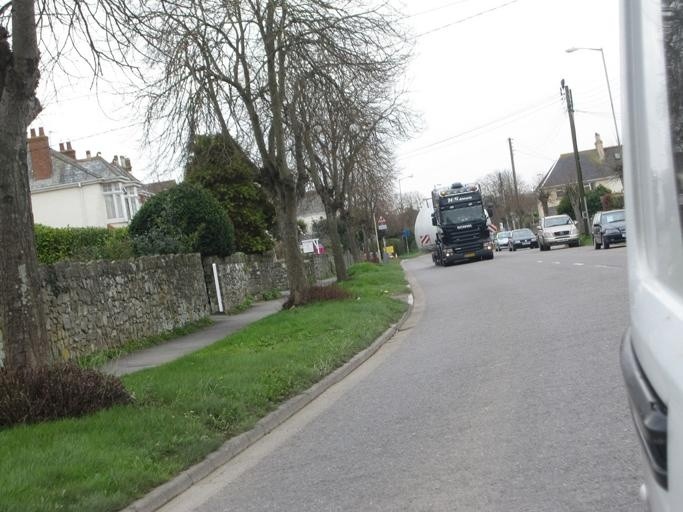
[536,213,579,251]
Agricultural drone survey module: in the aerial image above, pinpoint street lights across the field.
[565,46,618,159]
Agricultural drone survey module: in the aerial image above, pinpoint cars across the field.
[494,230,512,251]
[507,227,538,251]
[591,209,626,249]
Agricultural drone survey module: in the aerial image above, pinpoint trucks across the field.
[414,182,498,267]
